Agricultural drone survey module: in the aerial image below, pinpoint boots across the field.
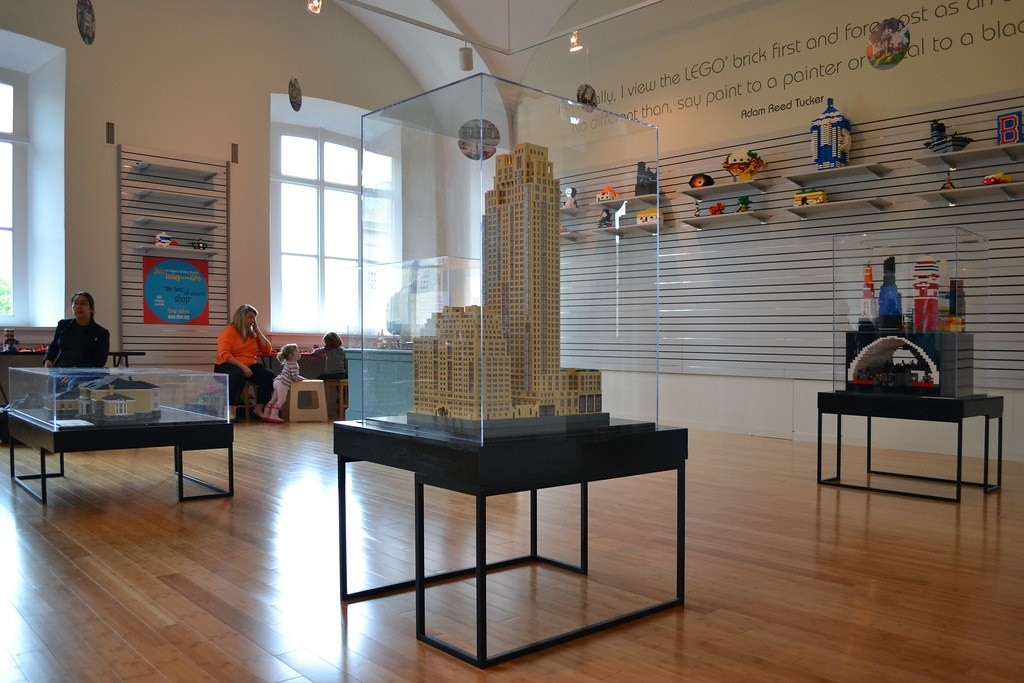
[262,403,272,421]
[269,407,285,422]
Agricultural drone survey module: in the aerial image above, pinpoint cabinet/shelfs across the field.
[558,142,1024,241]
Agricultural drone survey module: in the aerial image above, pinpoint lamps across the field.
[568,28,582,52]
[305,0,325,15]
[458,40,474,71]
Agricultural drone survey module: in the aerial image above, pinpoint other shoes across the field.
[230,418,237,422]
[251,411,262,419]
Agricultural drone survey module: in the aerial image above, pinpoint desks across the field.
[0,350,145,404]
[342,347,413,420]
[333,415,690,670]
[7,403,234,506]
[817,389,1005,504]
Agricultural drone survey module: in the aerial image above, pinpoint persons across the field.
[311,331,345,404]
[214,304,275,423]
[262,343,307,423]
[43,291,110,395]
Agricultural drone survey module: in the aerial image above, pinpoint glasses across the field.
[72,302,90,306]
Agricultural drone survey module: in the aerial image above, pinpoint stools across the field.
[325,379,348,420]
[241,381,257,420]
[287,378,328,423]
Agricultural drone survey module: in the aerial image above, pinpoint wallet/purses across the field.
[240,363,264,377]
